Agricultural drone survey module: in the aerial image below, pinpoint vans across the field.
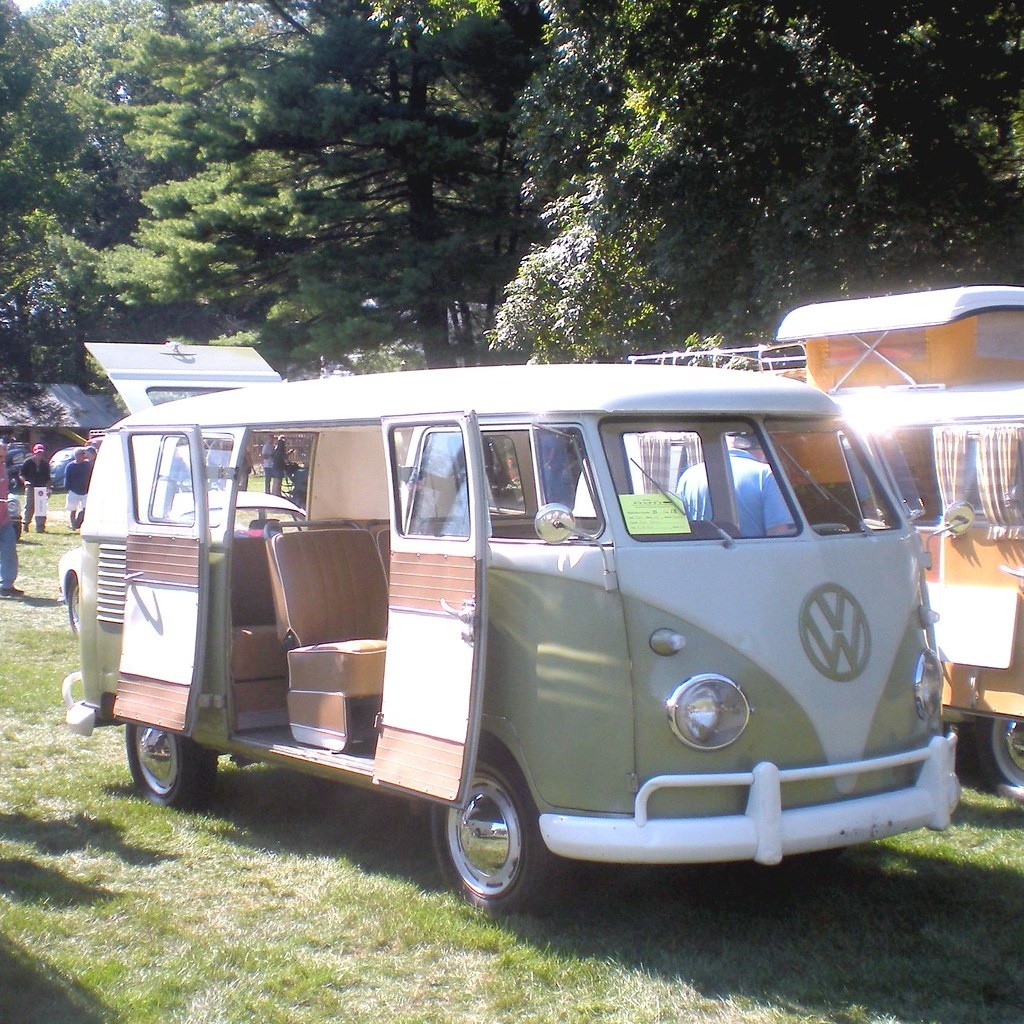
[55,344,961,910]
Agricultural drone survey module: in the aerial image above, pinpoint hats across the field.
[32,443,45,454]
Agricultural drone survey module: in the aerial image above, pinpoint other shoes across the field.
[0,587,25,599]
[23,523,29,533]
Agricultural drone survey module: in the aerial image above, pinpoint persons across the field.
[674,429,795,538]
[1,436,98,600]
[205,434,294,497]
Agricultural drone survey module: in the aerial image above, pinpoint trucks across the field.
[565,287,1024,807]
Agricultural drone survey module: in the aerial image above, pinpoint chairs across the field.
[233,515,390,754]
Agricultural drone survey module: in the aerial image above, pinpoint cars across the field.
[50,429,104,488]
